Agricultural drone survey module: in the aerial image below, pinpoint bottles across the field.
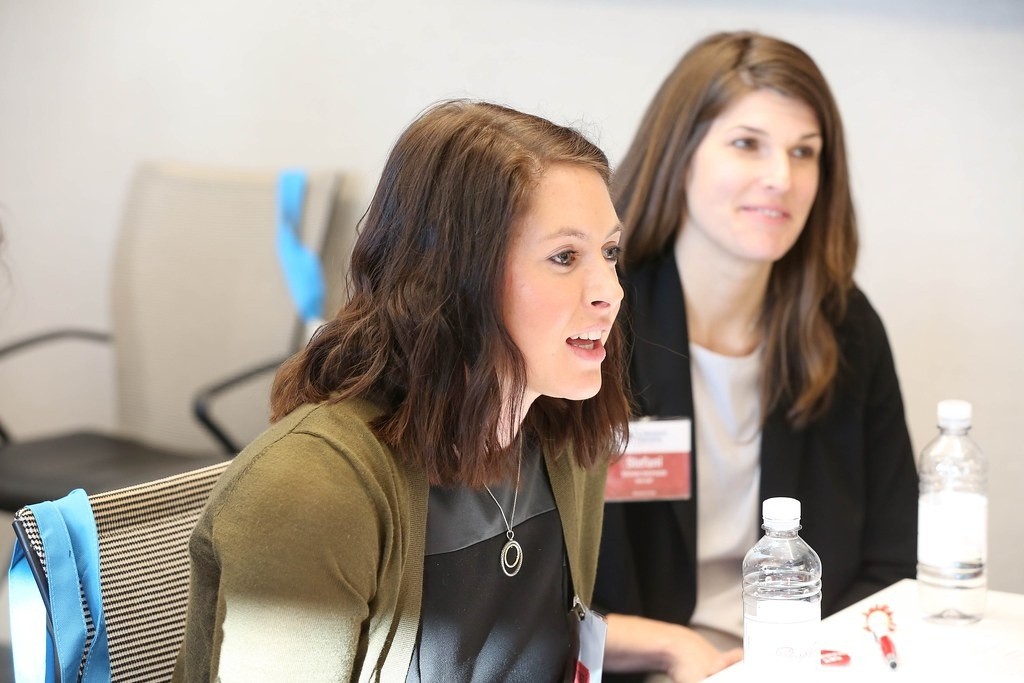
[916,399,988,628]
[741,496,822,683]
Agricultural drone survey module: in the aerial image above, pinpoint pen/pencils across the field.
[870,603,899,669]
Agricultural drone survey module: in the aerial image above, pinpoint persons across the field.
[590,30,919,682]
[170,97,624,682]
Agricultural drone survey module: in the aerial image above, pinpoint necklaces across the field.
[442,426,525,576]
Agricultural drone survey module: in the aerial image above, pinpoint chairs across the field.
[14,458,230,683]
[0,153,338,513]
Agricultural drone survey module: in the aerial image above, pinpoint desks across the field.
[698,577,1024,683]
